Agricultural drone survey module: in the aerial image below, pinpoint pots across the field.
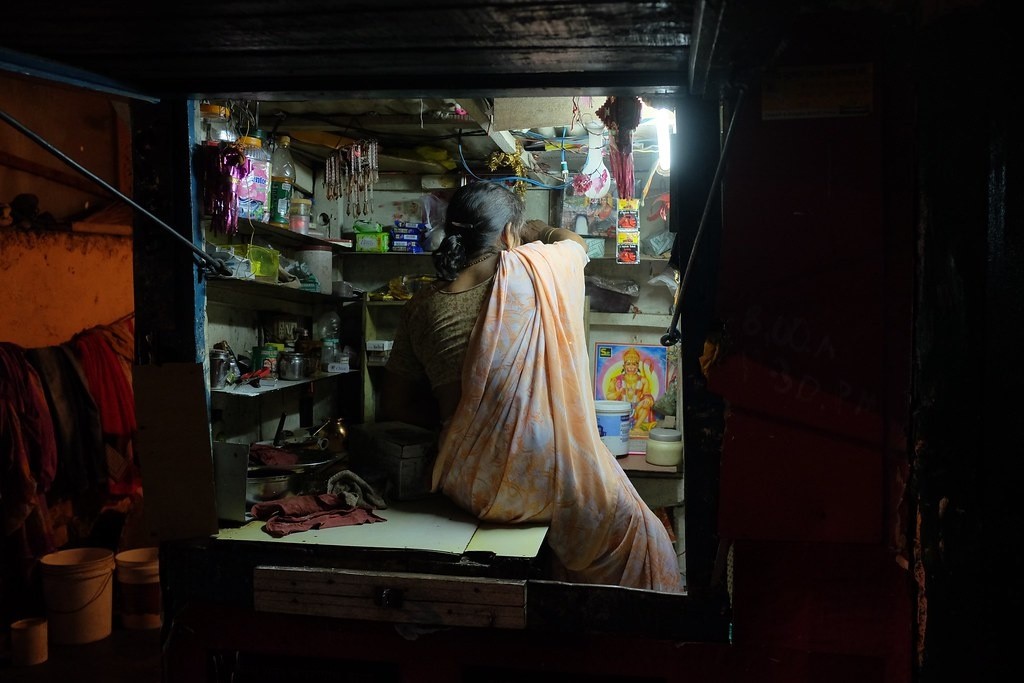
[247,466,291,502]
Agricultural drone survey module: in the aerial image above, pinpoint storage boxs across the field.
[351,422,447,501]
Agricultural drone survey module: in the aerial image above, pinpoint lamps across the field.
[654,103,670,179]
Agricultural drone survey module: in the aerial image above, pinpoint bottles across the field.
[294,329,317,375]
[645,429,682,466]
[328,416,346,452]
[290,198,312,235]
[209,349,227,390]
[272,136,296,229]
[294,243,333,295]
[321,342,334,372]
[237,136,268,221]
[316,302,342,359]
[278,351,305,381]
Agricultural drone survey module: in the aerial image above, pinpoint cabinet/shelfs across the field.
[198,211,365,404]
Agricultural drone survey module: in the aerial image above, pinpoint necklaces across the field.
[466,254,493,268]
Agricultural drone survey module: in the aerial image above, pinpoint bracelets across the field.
[544,227,558,243]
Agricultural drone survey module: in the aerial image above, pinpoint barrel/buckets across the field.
[594,400,631,456]
[40,547,115,644]
[11,618,48,665]
[115,547,162,630]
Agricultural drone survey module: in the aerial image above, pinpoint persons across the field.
[380,180,681,593]
